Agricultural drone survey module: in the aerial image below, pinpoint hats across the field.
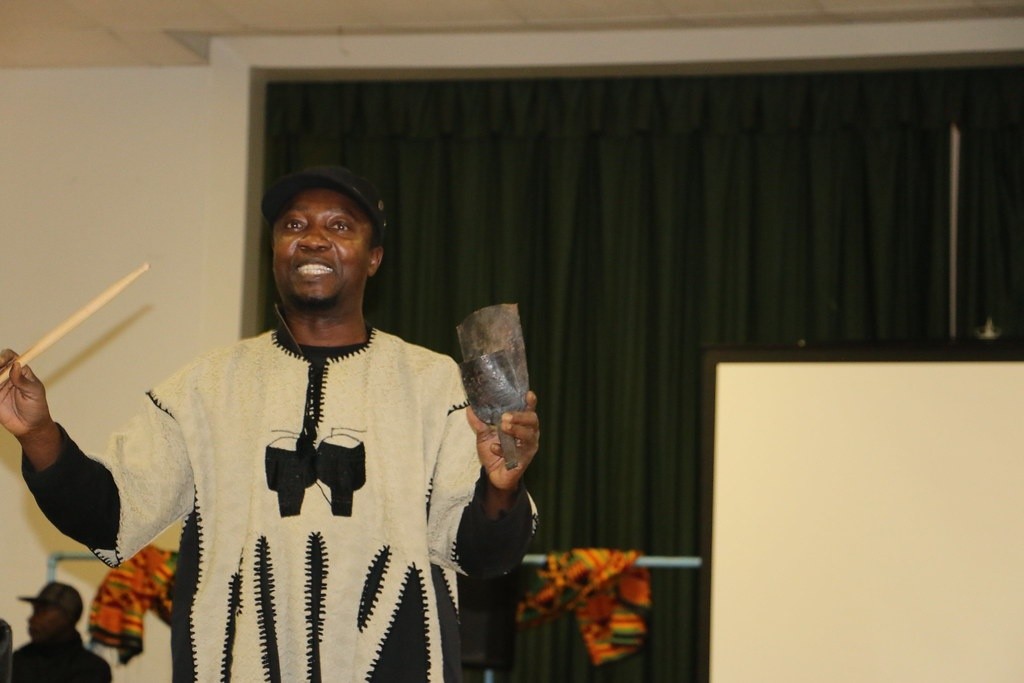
[260,165,389,239]
[16,581,85,623]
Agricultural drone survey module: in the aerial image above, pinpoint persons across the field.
[10,578,114,683]
[0,148,547,683]
